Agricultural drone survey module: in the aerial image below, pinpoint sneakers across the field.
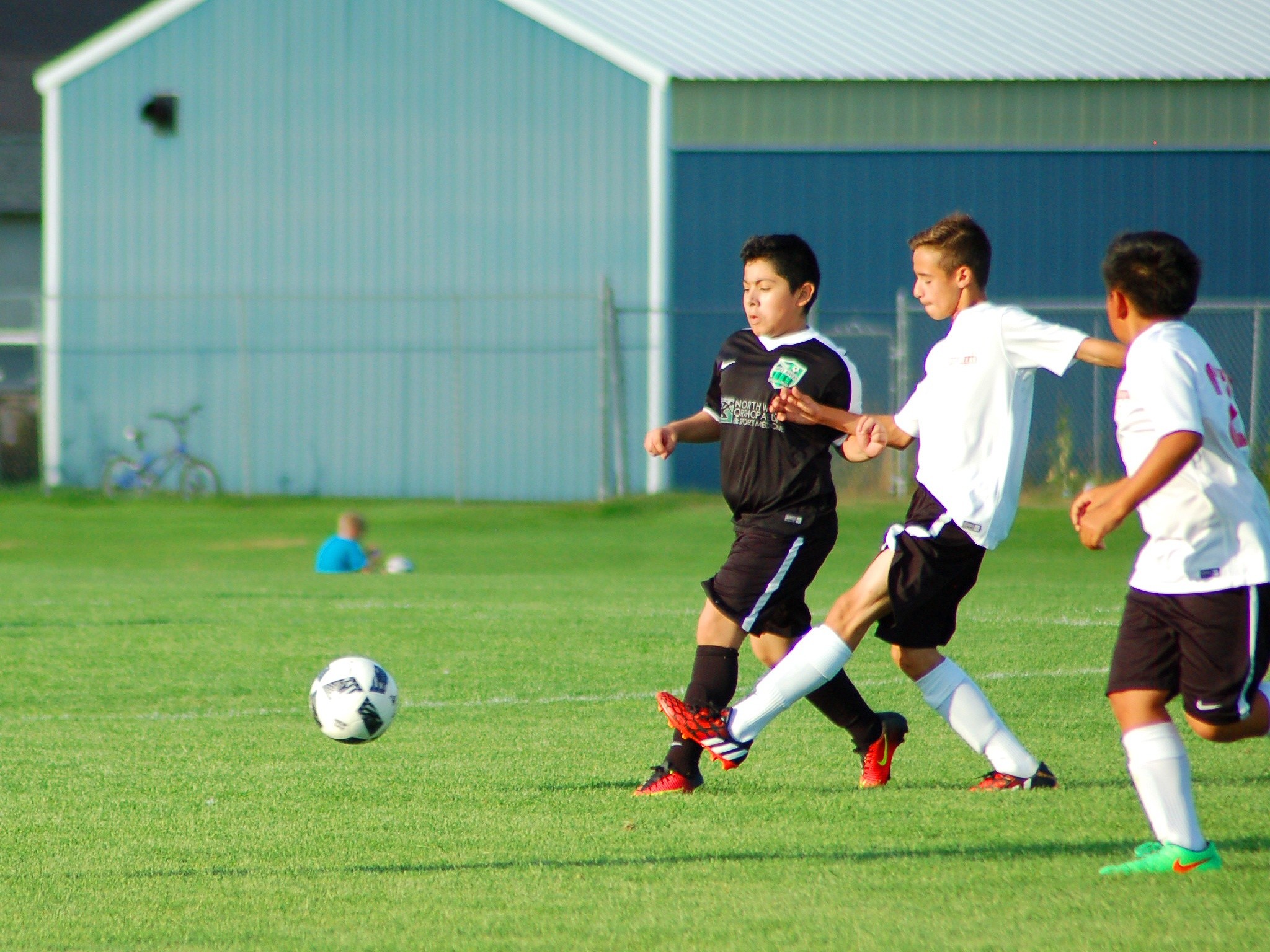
[852,710,910,789]
[632,763,696,797]
[1099,840,1225,877]
[655,692,754,772]
[969,762,1055,792]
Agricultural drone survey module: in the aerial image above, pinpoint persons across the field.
[311,512,377,575]
[1070,229,1270,876]
[654,212,1128,793]
[632,233,910,802]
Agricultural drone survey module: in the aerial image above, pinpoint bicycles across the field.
[98,402,222,500]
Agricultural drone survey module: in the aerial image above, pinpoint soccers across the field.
[307,656,402,747]
[384,554,417,575]
